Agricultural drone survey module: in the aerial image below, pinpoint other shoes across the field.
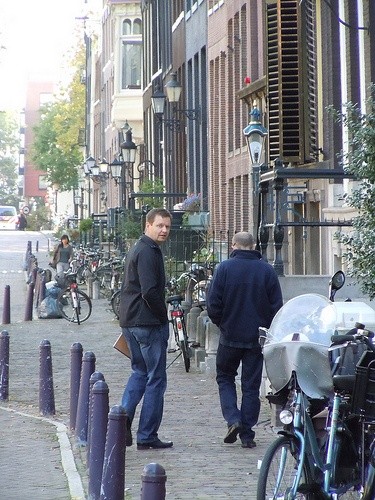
[241,439,256,448]
[223,421,242,443]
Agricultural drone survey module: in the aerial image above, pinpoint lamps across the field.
[243,100,269,197]
[74,72,199,190]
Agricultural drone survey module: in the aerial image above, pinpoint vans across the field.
[0,206,18,230]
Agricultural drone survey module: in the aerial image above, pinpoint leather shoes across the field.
[125,416,132,446]
[136,437,173,450]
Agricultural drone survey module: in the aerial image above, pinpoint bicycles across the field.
[70,244,126,317]
[54,259,92,326]
[165,272,197,372]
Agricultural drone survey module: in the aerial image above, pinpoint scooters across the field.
[256,272,375,500]
[177,249,216,311]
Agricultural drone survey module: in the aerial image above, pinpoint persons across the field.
[118,208,175,450]
[19,214,27,231]
[204,232,282,447]
[54,234,73,273]
